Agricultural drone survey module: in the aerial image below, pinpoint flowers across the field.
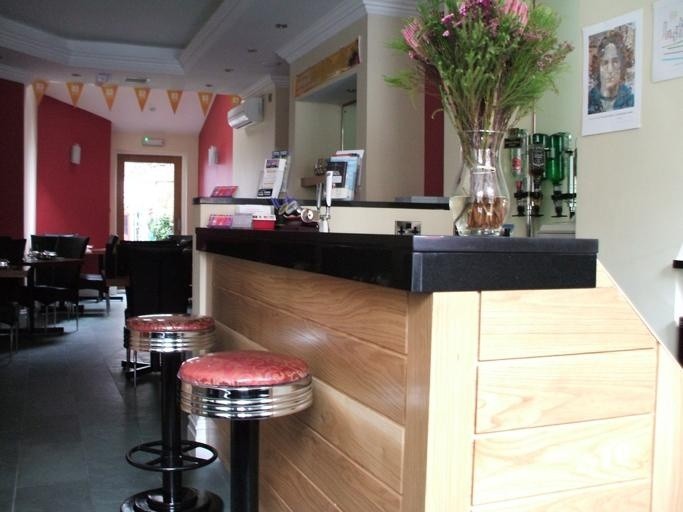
[385,0,572,136]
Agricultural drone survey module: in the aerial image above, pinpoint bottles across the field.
[508,126,578,219]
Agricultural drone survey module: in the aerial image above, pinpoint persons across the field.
[586,30,634,114]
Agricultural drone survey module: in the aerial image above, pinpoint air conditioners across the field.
[227,96,264,130]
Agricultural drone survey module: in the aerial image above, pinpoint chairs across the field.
[0,233,118,363]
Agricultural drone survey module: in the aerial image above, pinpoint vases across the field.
[445,137,513,241]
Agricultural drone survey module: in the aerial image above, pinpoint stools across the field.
[119,312,312,512]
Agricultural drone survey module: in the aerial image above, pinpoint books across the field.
[259,157,287,198]
[328,155,357,201]
[334,148,365,187]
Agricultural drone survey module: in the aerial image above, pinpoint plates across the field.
[31,250,57,260]
